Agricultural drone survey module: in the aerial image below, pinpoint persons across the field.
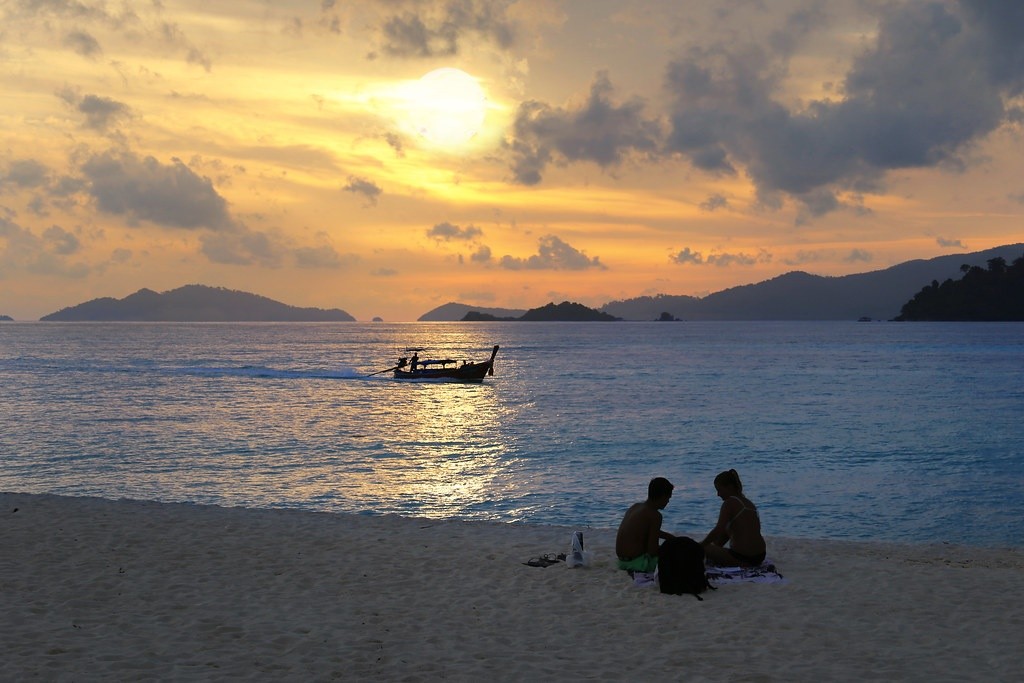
[616,477,675,572]
[701,469,766,567]
[409,353,419,371]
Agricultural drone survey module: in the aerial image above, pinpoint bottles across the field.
[573,532,583,565]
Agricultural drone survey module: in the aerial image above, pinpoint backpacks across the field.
[656,536,719,601]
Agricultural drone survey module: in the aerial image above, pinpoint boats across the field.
[394,344,500,383]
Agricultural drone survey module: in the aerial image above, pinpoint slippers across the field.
[521,557,552,567]
[542,553,560,563]
